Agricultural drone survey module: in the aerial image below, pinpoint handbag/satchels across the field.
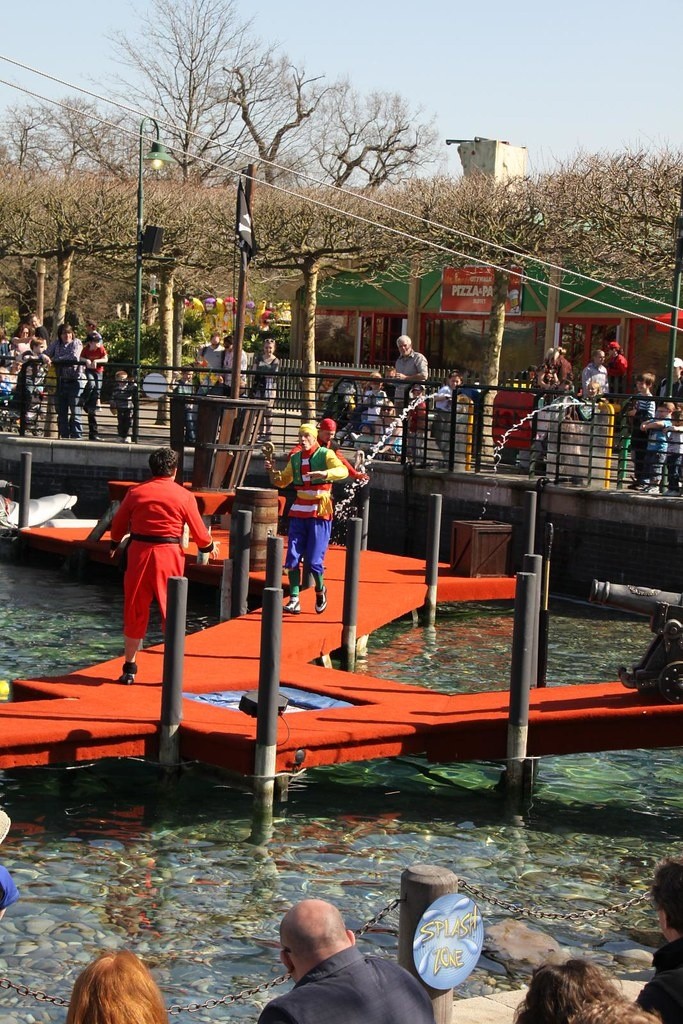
[430,415,440,438]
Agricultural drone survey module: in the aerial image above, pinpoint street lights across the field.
[127,116,176,443]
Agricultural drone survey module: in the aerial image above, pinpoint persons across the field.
[289,418,370,569]
[264,423,350,614]
[111,371,138,445]
[393,335,428,416]
[0,312,109,443]
[172,332,248,445]
[625,358,683,496]
[363,371,388,425]
[511,957,621,1024]
[408,383,427,464]
[635,855,683,1024]
[107,446,222,691]
[250,338,280,443]
[382,368,396,404]
[373,401,402,462]
[431,368,464,463]
[566,995,664,1024]
[514,340,629,485]
[352,420,374,472]
[256,899,435,1024]
[66,949,170,1024]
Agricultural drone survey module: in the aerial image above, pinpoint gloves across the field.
[353,473,369,480]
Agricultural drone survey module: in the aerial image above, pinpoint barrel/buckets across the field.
[229,486,279,572]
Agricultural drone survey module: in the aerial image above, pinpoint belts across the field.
[130,534,179,543]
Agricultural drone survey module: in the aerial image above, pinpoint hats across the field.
[674,358,683,368]
[359,420,375,432]
[299,423,318,439]
[84,331,103,343]
[320,418,336,430]
[608,342,619,348]
[411,384,422,392]
[0,811,11,845]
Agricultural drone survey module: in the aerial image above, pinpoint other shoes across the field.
[638,486,660,495]
[663,489,681,496]
[120,665,136,684]
[628,485,636,489]
[257,436,271,443]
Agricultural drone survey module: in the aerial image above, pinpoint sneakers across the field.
[283,601,300,614]
[314,586,328,614]
